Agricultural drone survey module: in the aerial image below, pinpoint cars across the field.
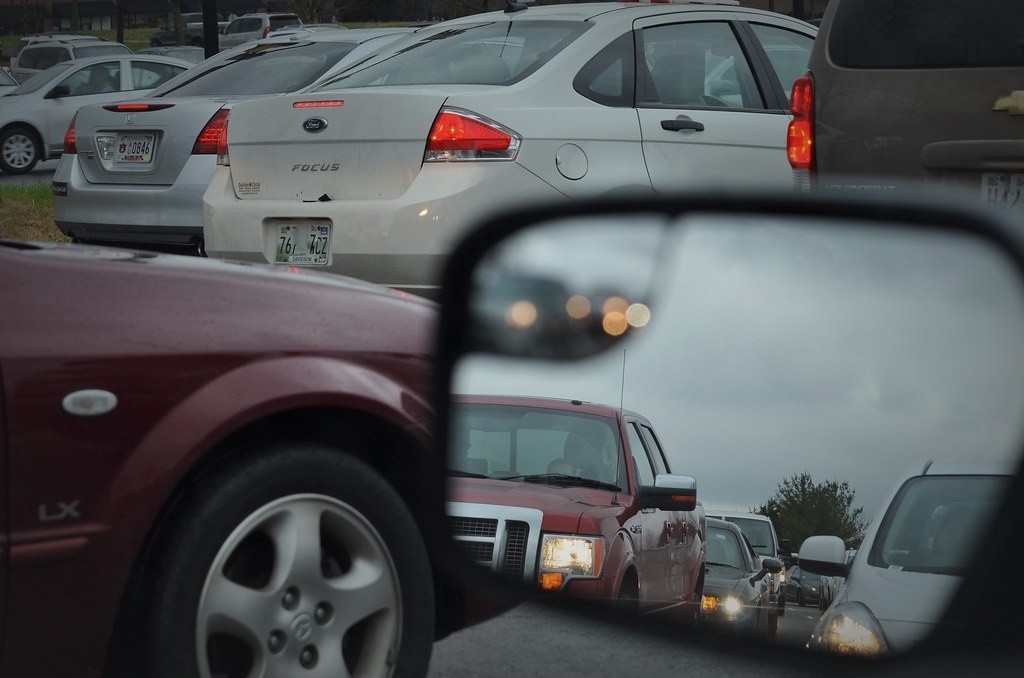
[202,1,819,299]
[1,12,808,259]
[689,459,1022,652]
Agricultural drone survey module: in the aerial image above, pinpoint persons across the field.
[548,420,613,482]
[911,503,981,568]
[72,65,115,97]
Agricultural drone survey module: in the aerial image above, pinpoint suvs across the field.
[0,239,519,678]
[445,394,707,637]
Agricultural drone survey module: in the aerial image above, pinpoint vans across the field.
[785,2,1023,221]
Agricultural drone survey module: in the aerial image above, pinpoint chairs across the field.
[646,56,704,106]
